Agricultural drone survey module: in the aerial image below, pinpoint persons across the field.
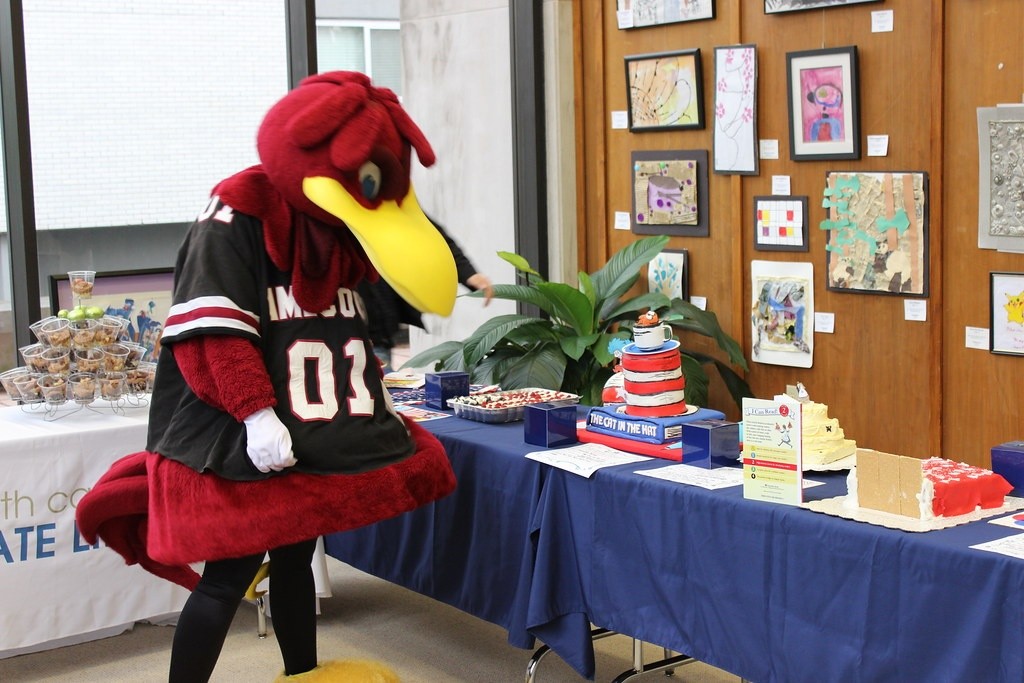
[421,208,495,307]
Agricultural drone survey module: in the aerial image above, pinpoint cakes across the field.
[792,381,856,465]
[450,387,577,410]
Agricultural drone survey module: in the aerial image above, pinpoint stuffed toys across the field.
[75,72,459,683]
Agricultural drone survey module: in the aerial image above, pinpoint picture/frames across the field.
[784,45,860,162]
[989,271,1024,357]
[622,47,705,133]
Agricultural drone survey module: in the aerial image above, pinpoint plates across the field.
[622,339,680,355]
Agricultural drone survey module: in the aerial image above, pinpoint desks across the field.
[324,374,618,683]
[526,451,1024,683]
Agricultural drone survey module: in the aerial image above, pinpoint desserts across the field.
[0,271,158,404]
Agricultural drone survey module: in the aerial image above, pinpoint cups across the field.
[0,271,157,404]
[633,321,673,352]
